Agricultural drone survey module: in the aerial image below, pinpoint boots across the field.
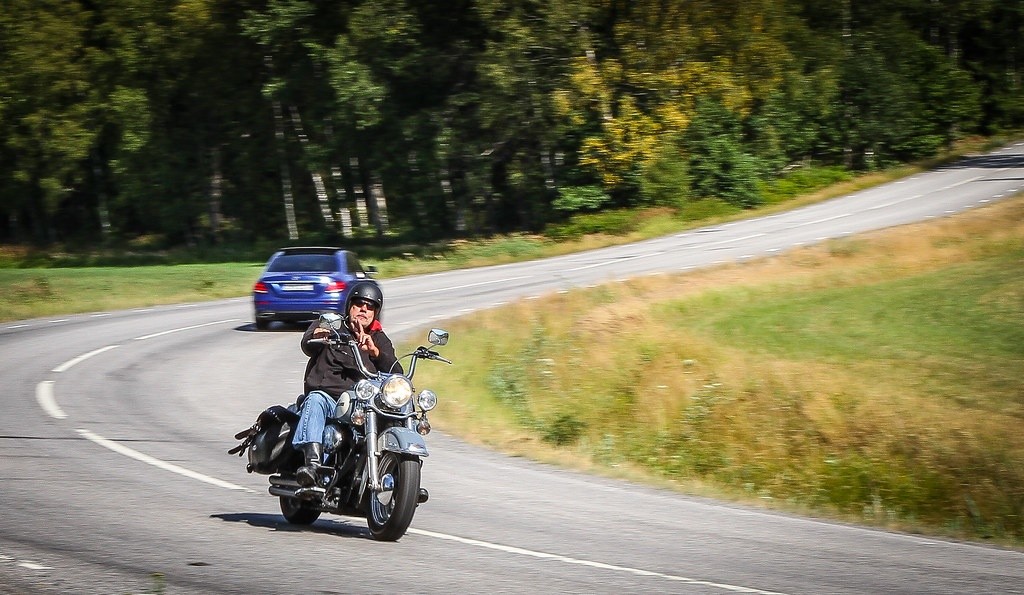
[295,442,322,486]
[417,488,428,504]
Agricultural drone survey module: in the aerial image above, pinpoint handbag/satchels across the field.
[227,405,303,475]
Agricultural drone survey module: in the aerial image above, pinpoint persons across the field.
[297,282,429,503]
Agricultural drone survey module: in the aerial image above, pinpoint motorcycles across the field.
[227,314,453,544]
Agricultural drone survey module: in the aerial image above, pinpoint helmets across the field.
[344,282,382,320]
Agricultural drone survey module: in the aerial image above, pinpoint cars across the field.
[251,246,381,335]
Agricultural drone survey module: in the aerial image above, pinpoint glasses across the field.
[348,298,377,312]
